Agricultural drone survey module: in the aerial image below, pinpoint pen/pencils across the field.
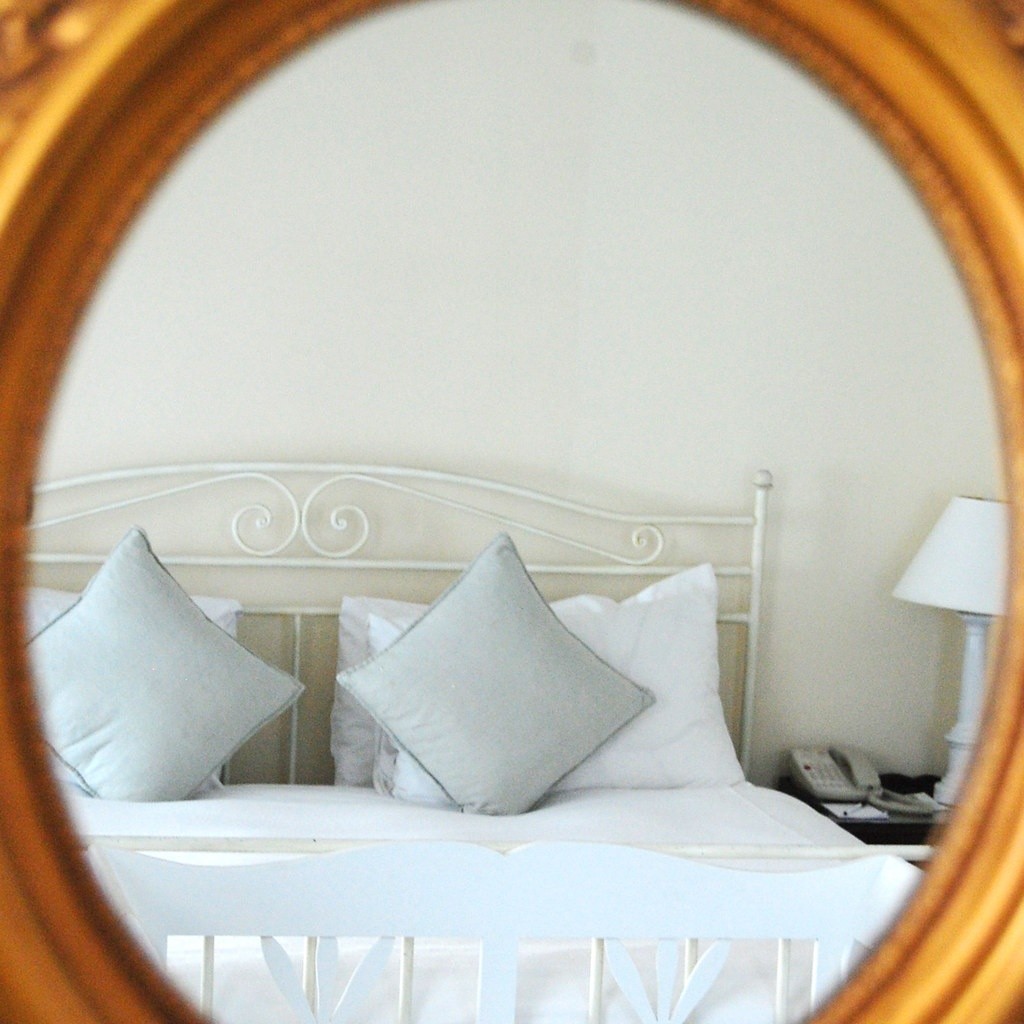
[843,801,866,815]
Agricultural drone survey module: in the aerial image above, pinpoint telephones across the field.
[785,740,884,801]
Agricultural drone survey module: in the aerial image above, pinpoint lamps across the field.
[895,494,1007,805]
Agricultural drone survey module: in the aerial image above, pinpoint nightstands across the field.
[775,765,947,867]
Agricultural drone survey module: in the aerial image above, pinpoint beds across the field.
[28,456,922,1024]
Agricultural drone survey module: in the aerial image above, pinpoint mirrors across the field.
[0,0,1024,1024]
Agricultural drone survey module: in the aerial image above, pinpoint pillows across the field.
[28,588,242,803]
[27,525,304,803]
[335,533,652,819]
[368,566,745,797]
[338,593,616,787]
